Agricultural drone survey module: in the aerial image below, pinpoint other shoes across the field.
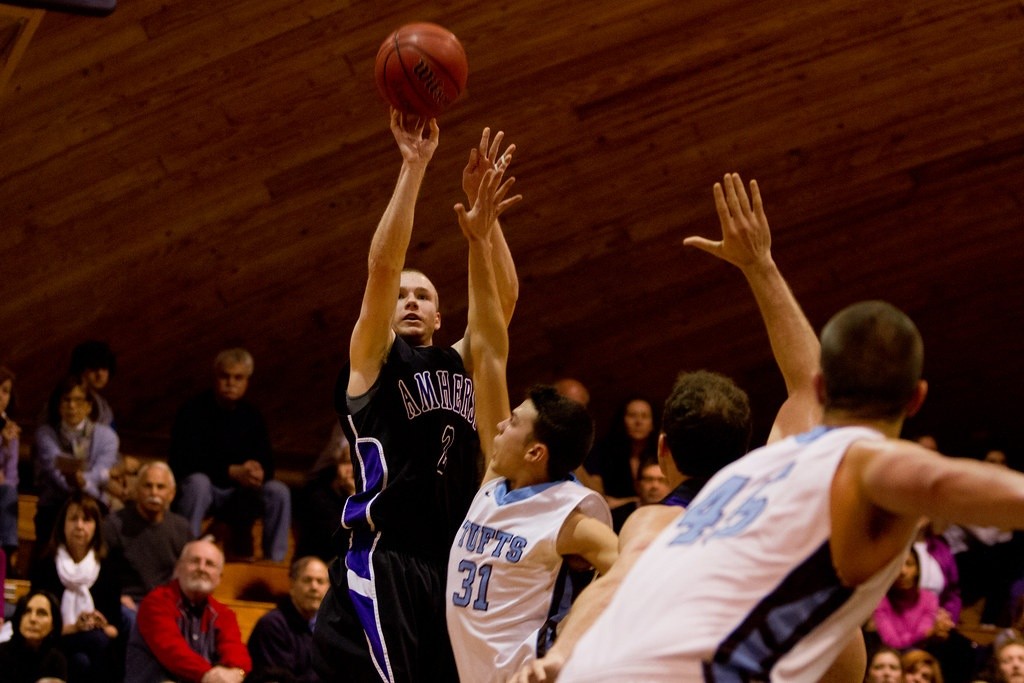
[3,545,22,579]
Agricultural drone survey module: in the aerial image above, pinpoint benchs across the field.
[4,447,308,645]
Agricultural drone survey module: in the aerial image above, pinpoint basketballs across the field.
[374,22,468,118]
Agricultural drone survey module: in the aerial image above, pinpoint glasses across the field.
[59,397,87,408]
[640,475,664,480]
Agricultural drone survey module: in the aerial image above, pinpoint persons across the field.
[446,167,620,683]
[0,338,1023,682]
[337,101,521,683]
[553,299,1023,683]
[512,168,828,683]
